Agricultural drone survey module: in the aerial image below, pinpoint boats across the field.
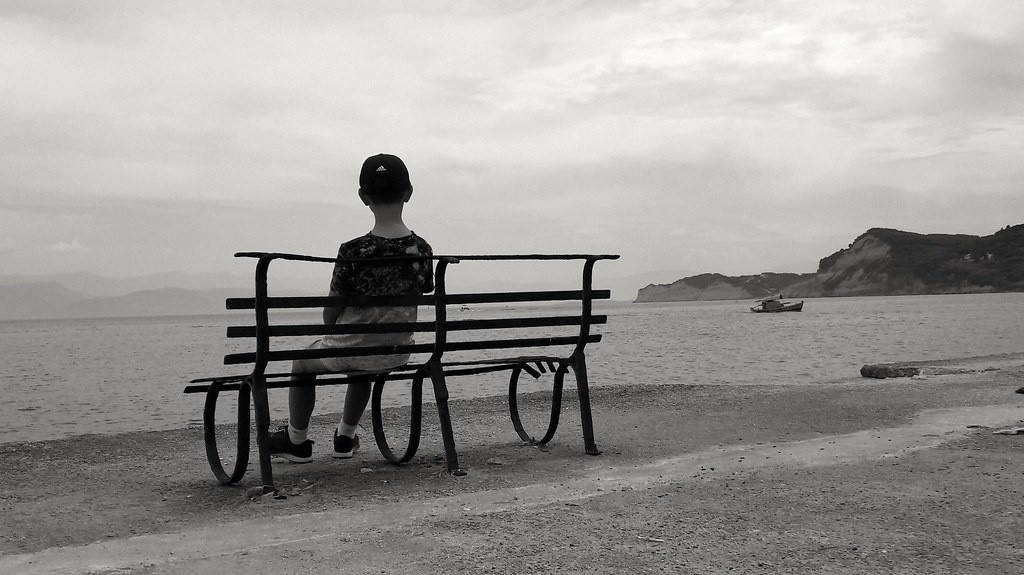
[750,295,803,311]
[460,305,469,313]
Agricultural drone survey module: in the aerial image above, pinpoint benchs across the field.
[183,252,620,496]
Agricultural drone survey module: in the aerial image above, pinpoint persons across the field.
[256,153,434,463]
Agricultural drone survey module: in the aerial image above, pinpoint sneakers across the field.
[331,427,361,458]
[256,425,317,463]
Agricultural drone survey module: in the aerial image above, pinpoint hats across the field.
[359,152,409,197]
